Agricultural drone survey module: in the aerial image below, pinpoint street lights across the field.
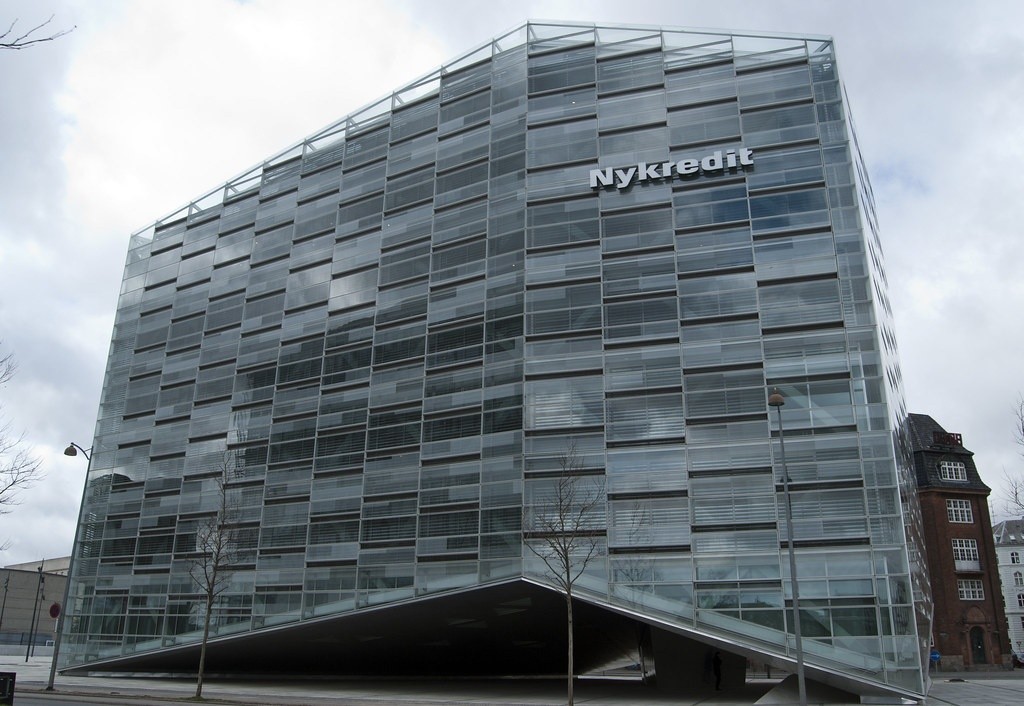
[768,387,808,706]
[47,441,95,690]
